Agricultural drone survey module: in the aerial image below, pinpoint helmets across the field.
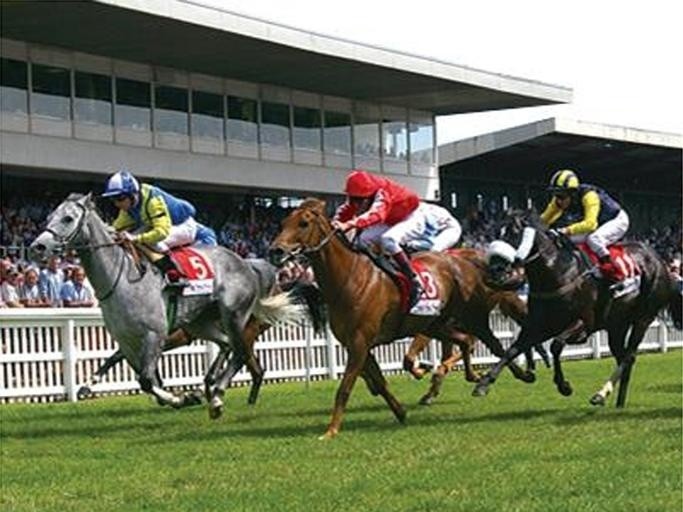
[545,170,580,193]
[344,171,378,197]
[101,170,140,197]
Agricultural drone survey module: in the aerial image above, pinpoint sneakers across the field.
[167,269,180,284]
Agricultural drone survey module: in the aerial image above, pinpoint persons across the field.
[1,180,285,309]
[101,169,217,302]
[539,169,641,302]
[452,191,683,297]
[330,171,465,315]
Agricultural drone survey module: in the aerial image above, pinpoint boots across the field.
[598,255,626,291]
[391,251,424,306]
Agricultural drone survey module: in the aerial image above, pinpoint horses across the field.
[471,201,683,408]
[76,280,330,405]
[263,196,537,442]
[402,249,551,406]
[27,191,312,419]
